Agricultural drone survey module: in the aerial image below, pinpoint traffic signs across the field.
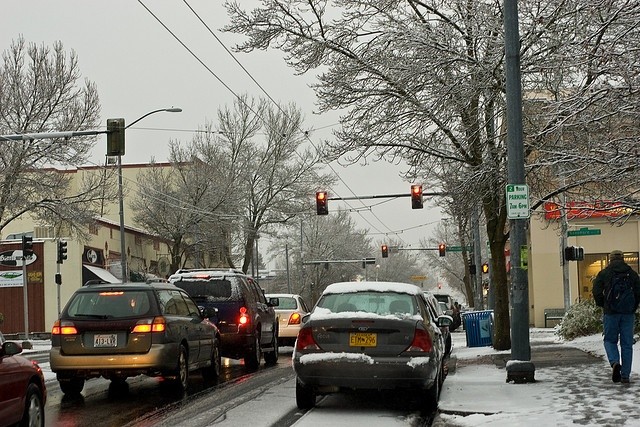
[568,226,602,237]
[388,245,398,253]
[446,246,472,252]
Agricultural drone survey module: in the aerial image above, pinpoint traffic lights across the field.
[411,185,423,209]
[482,264,489,272]
[382,245,388,257]
[438,285,441,289]
[564,246,584,261]
[61,240,67,263]
[25,236,33,257]
[316,192,328,215]
[439,244,445,256]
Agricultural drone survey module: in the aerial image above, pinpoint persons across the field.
[592,249,640,383]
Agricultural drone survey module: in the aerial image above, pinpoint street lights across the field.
[116,106,183,282]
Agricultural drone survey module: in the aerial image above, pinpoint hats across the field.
[610,250,624,260]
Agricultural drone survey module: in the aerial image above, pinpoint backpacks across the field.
[605,268,635,313]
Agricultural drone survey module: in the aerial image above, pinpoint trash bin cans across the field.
[462,310,494,348]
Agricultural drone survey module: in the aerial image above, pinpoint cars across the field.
[292,281,453,413]
[264,293,311,347]
[423,290,463,357]
[0,331,47,427]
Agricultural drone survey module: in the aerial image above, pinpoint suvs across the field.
[166,267,279,369]
[50,278,222,394]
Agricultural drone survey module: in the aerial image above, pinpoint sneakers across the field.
[621,378,629,383]
[612,363,621,383]
